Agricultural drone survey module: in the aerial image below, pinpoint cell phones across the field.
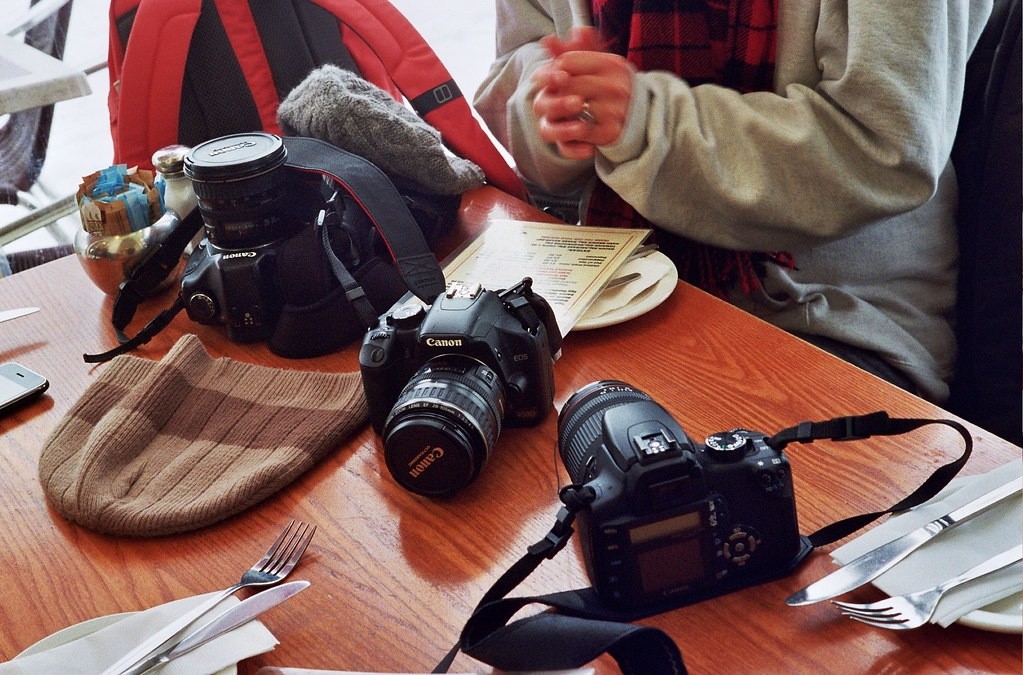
[0,363,50,417]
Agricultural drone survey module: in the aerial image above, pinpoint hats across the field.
[36,332,371,532]
[276,66,486,194]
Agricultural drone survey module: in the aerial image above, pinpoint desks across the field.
[1,30,94,127]
[0,166,1023,675]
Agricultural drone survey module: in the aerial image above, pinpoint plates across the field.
[834,514,1023,633]
[9,609,237,675]
[565,243,679,335]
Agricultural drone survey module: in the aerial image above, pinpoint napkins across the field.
[579,255,669,321]
[826,453,1023,627]
[0,590,281,675]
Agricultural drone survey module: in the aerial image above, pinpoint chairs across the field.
[0,0,73,276]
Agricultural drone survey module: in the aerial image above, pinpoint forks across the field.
[831,540,1022,630]
[117,519,318,674]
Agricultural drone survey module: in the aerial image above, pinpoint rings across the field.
[574,98,595,124]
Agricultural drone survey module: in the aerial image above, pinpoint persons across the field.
[468,0,998,407]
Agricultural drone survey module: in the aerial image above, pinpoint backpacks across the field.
[107,0,530,201]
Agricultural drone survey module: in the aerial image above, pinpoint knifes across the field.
[119,581,311,675]
[784,474,1022,612]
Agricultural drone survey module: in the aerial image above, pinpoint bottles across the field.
[154,143,204,246]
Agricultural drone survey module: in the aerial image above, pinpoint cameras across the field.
[180,133,376,345]
[358,274,555,499]
[555,380,799,618]
